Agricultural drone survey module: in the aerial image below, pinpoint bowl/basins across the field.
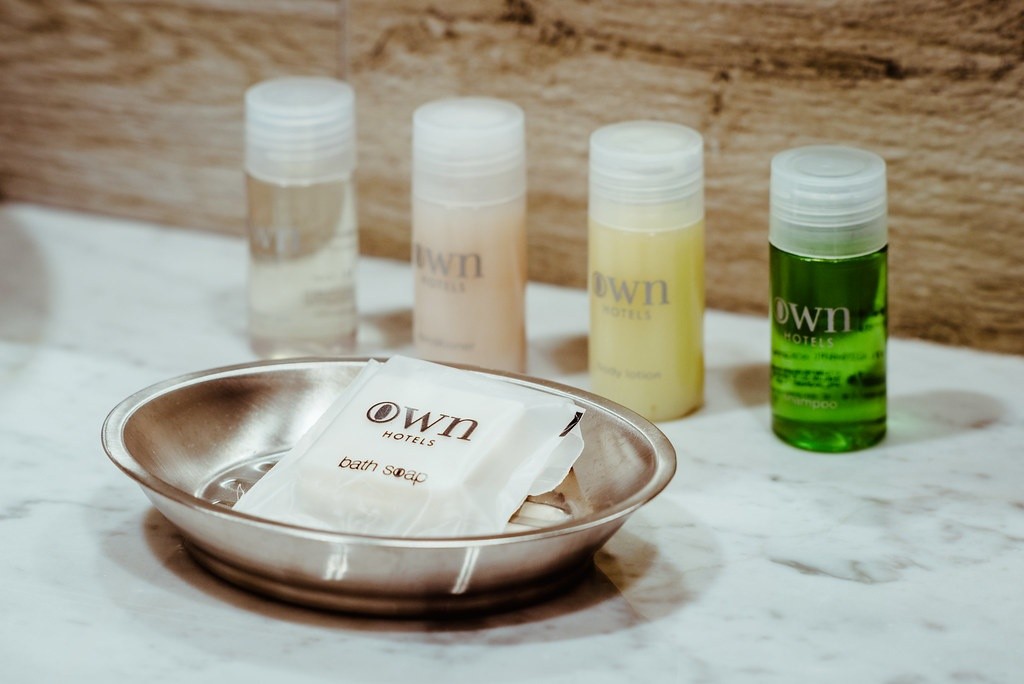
[99,353,680,616]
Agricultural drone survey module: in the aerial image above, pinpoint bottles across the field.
[769,143,888,452]
[587,119,706,425]
[410,98,525,376]
[241,73,362,360]
